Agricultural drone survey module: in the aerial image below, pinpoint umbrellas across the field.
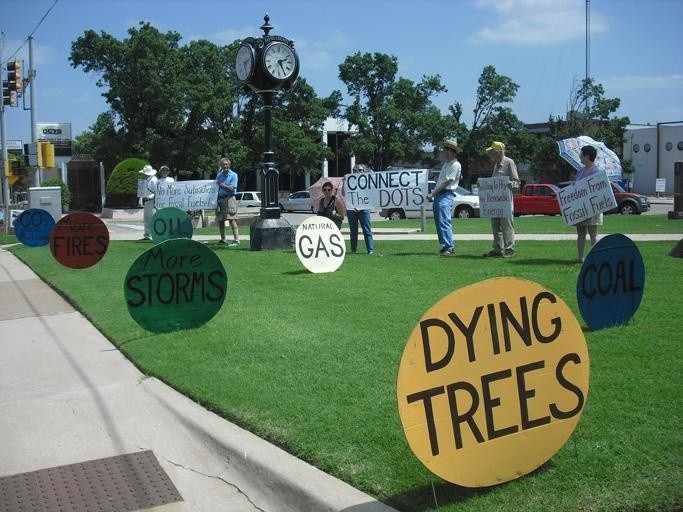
[555,136,624,182]
[307,176,346,216]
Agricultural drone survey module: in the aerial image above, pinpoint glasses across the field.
[322,188,331,192]
[437,146,450,152]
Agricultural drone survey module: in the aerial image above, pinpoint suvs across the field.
[558,180,651,216]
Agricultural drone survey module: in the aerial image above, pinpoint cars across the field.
[379,180,480,221]
[233,190,261,207]
[278,191,319,214]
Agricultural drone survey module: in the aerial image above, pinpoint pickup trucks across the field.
[513,183,563,218]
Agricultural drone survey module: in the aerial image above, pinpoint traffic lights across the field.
[2,79,14,106]
[6,58,20,91]
[12,161,21,175]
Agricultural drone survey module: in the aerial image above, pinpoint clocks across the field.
[235,12,299,250]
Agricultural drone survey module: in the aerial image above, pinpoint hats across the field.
[138,165,156,177]
[485,141,505,152]
[436,135,463,154]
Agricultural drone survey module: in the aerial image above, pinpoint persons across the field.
[214,156,240,246]
[475,140,522,257]
[156,164,175,181]
[346,163,376,254]
[425,137,463,255]
[571,145,603,263]
[316,181,344,231]
[136,165,158,241]
